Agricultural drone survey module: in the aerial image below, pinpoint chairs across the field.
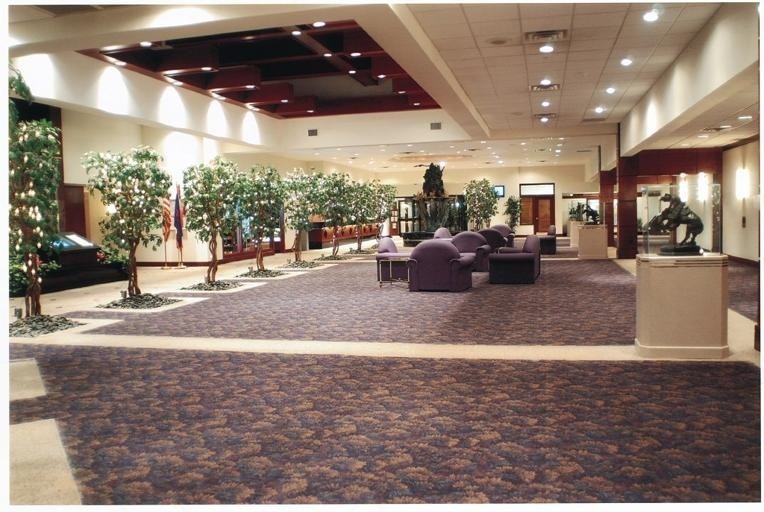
[375,224,556,293]
